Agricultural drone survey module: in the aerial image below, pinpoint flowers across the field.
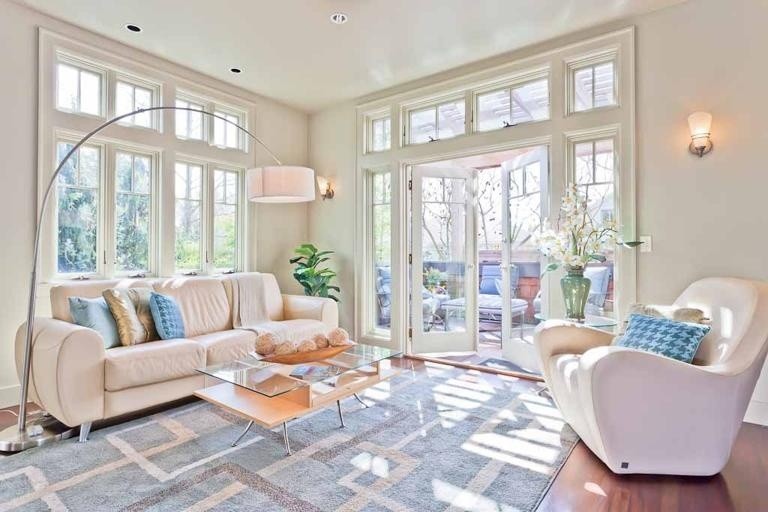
[530,179,643,269]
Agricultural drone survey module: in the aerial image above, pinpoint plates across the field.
[257,338,358,365]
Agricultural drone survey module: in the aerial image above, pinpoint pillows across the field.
[102,281,158,345]
[612,299,707,325]
[607,311,713,360]
[68,294,120,347]
[149,289,188,342]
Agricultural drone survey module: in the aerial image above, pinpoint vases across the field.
[559,268,594,326]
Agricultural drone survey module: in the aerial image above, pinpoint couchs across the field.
[481,264,519,325]
[526,274,761,479]
[378,268,449,332]
[11,272,340,446]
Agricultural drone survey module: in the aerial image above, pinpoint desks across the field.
[442,294,529,343]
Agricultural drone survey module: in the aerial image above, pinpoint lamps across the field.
[316,173,335,201]
[684,109,715,159]
[3,100,316,453]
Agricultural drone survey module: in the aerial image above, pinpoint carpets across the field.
[0,358,584,512]
